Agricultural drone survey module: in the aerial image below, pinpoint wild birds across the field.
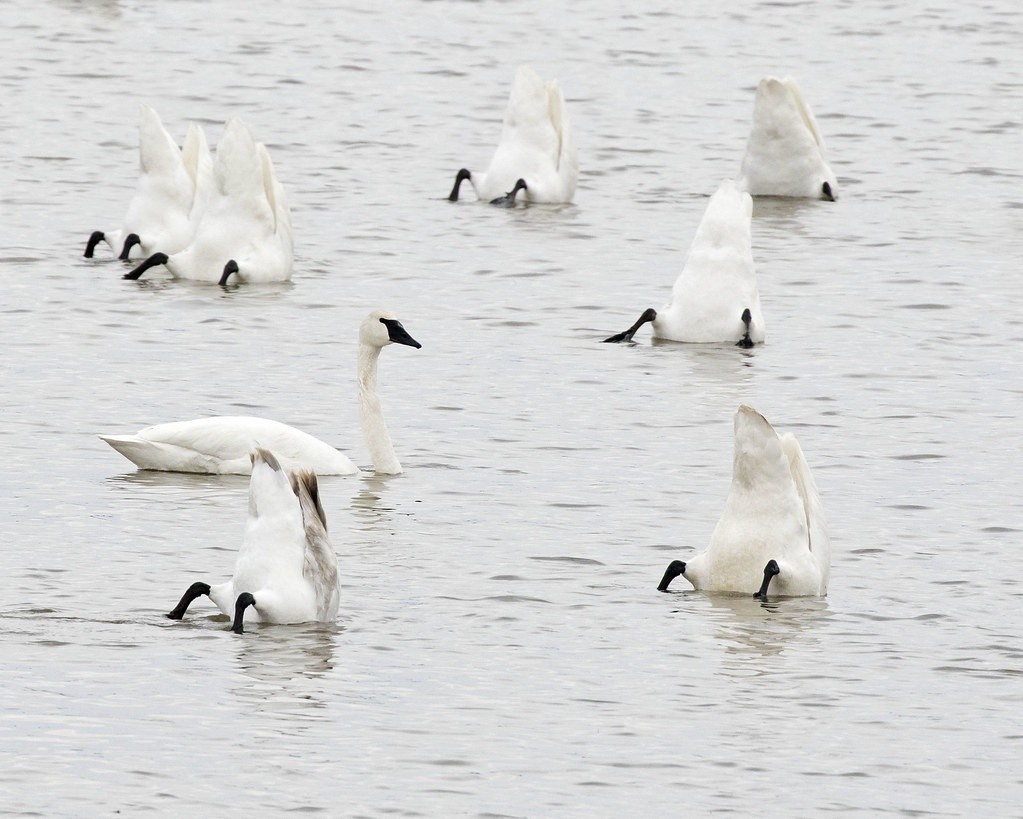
[602,177,765,348]
[448,65,579,207]
[742,77,842,201]
[166,435,341,635]
[659,403,830,599]
[98,310,423,478]
[84,103,295,287]
